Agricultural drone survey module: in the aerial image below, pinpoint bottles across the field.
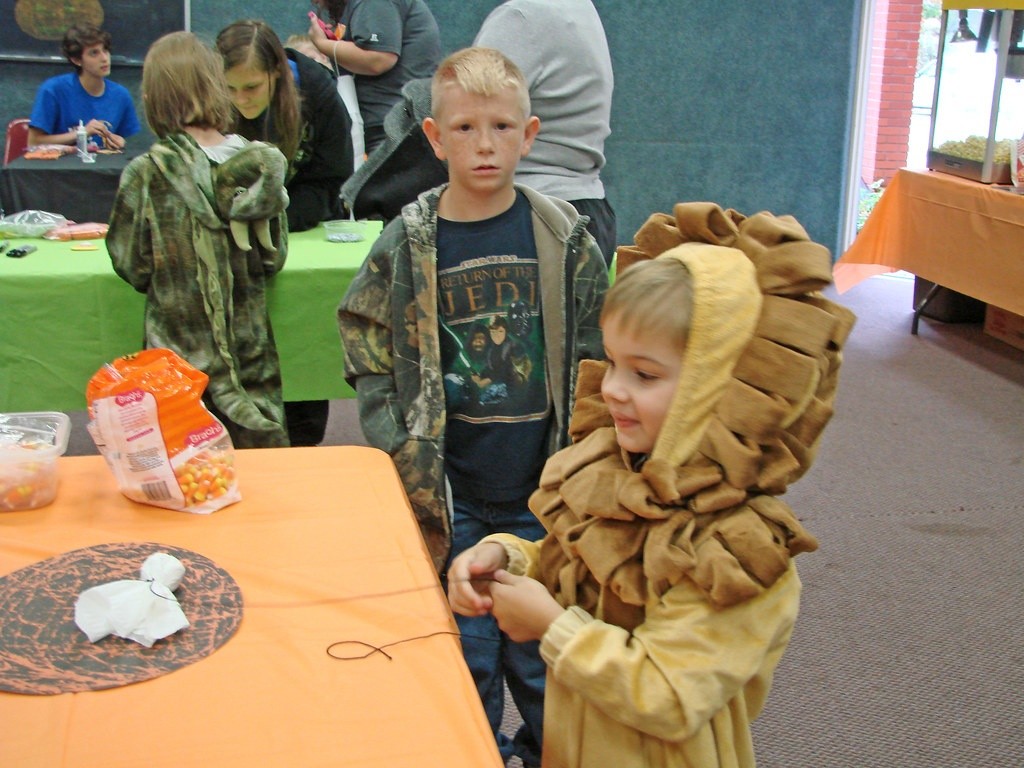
[76,118,88,157]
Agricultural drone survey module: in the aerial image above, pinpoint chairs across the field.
[3,118,31,167]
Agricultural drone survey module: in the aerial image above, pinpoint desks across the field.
[0,147,150,227]
[0,445,505,766]
[0,220,388,414]
[897,166,1024,335]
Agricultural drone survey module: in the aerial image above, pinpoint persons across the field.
[27,23,141,151]
[284,34,328,68]
[105,31,291,449]
[308,0,440,161]
[447,200,856,767]
[336,48,611,768]
[215,21,355,233]
[338,0,617,273]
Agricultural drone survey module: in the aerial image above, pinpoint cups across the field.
[82,153,97,163]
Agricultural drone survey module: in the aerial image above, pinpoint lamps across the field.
[949,10,979,44]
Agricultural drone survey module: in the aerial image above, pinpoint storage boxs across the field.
[926,150,1012,185]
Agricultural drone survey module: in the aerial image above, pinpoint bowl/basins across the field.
[323,220,367,243]
[0,411,73,513]
